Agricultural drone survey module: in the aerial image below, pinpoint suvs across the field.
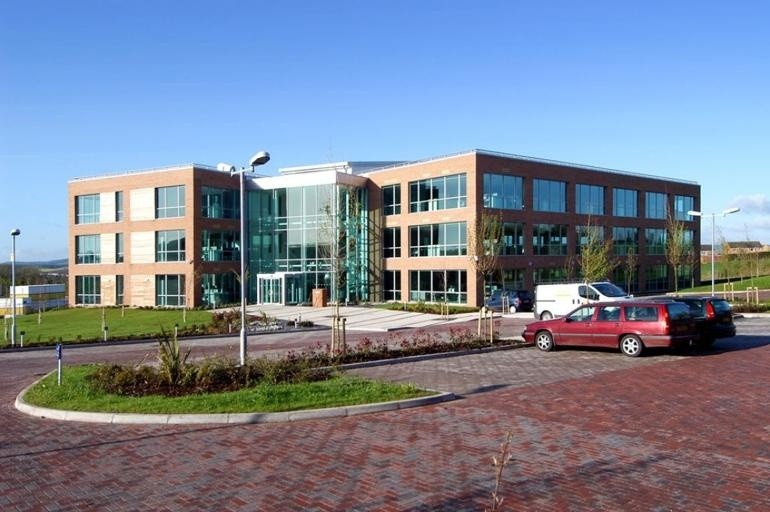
[521,299,696,357]
[485,288,530,311]
[645,296,737,352]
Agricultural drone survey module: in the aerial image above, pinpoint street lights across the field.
[216,151,272,365]
[10,226,21,347]
[686,207,740,298]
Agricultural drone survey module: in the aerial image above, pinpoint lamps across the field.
[474,255,478,261]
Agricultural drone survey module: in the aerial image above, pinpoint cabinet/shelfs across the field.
[312,288,326,307]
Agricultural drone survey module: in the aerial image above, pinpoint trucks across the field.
[535,283,633,318]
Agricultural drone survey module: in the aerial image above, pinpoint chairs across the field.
[596,310,610,321]
[611,308,620,319]
